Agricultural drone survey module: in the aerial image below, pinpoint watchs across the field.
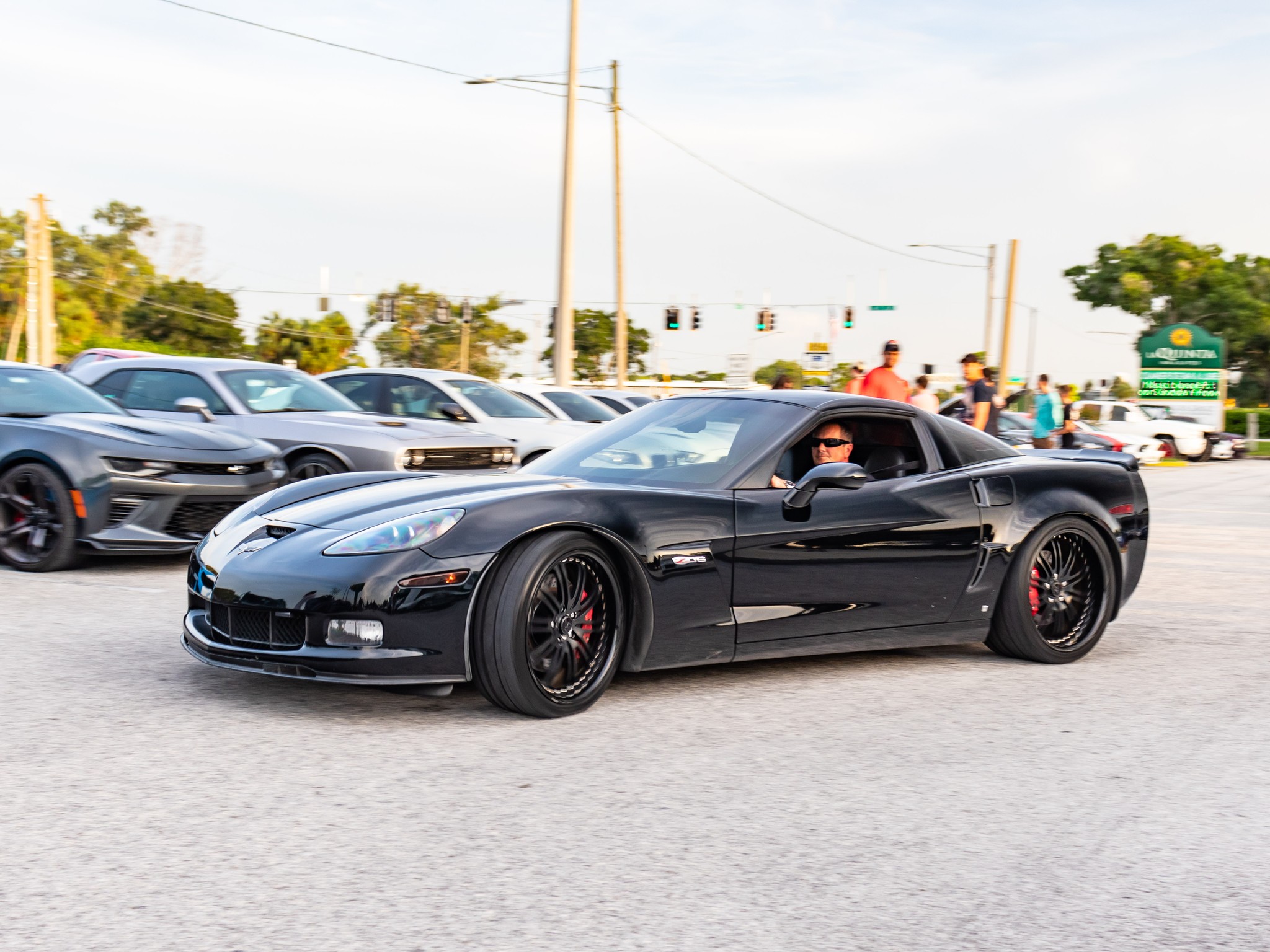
[784,480,795,488]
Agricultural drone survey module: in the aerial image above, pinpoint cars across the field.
[64,355,524,483]
[185,385,1151,721]
[0,355,296,573]
[496,383,727,473]
[315,364,680,475]
[587,390,660,416]
[935,398,1248,463]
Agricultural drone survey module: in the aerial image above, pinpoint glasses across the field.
[810,437,851,448]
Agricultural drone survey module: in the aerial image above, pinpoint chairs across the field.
[862,445,915,481]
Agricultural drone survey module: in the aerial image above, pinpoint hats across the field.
[885,340,899,352]
[961,353,980,363]
[916,376,927,382]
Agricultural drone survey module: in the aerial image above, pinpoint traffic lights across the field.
[692,310,700,329]
[667,309,679,330]
[844,308,853,327]
[757,310,775,330]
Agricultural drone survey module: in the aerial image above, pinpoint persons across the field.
[910,375,940,414]
[772,375,794,389]
[1034,374,1065,449]
[844,366,866,395]
[861,340,908,403]
[1050,385,1080,449]
[770,418,875,488]
[960,353,1007,437]
